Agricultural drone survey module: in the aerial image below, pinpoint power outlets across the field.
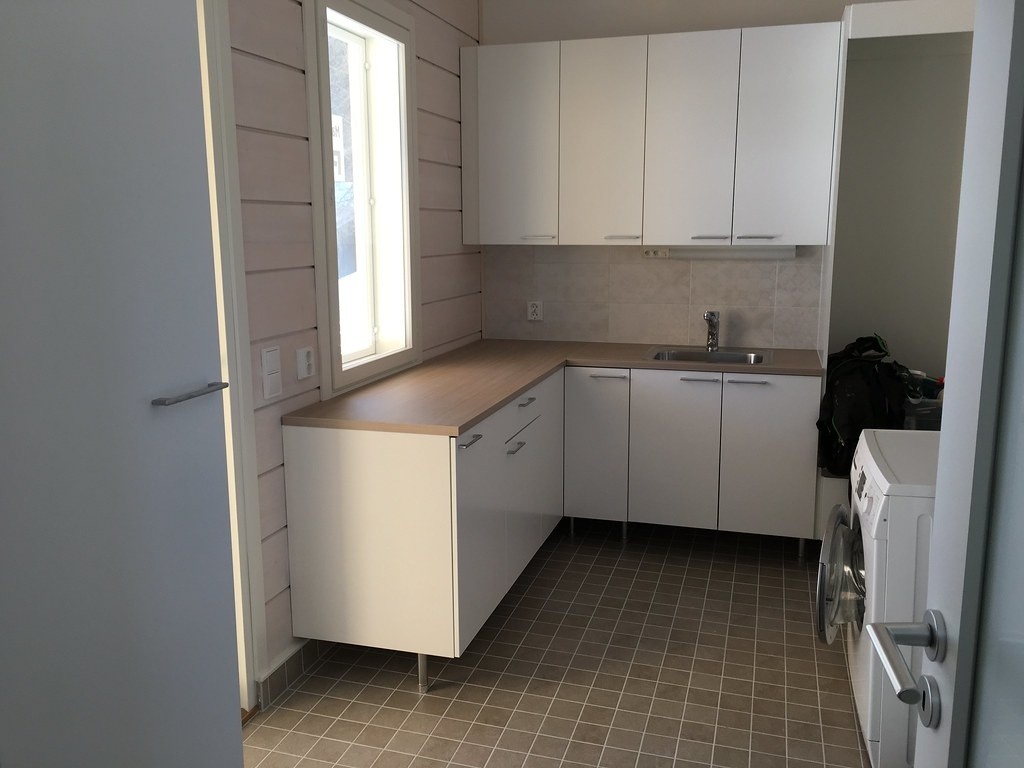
[526,301,543,321]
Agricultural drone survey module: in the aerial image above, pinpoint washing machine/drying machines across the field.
[817,428,941,766]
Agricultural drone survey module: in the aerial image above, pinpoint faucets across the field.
[703,310,720,351]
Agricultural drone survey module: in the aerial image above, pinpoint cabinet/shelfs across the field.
[627,368,822,558]
[458,35,649,248]
[563,365,627,539]
[280,365,564,693]
[641,20,841,245]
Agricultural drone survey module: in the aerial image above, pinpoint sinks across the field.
[653,351,764,363]
